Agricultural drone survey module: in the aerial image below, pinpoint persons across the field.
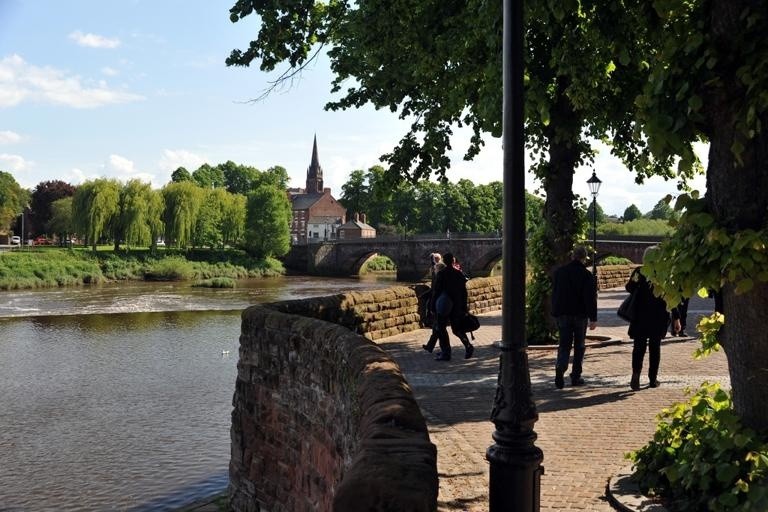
[551,247,597,389]
[424,251,474,360]
[625,246,681,391]
[672,288,689,337]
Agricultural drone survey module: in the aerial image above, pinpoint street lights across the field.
[403,215,408,240]
[586,166,602,300]
[16,212,25,249]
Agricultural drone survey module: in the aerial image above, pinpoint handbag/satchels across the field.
[434,290,454,319]
[617,294,631,324]
[462,311,480,333]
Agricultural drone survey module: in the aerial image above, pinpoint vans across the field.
[11,235,20,244]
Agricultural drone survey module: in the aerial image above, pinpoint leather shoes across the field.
[423,344,433,354]
[433,352,452,361]
[464,345,475,359]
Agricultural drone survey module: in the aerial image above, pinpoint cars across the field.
[156,239,165,247]
[27,237,77,245]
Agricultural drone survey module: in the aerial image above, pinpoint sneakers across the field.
[648,374,660,388]
[630,370,641,391]
[671,330,688,337]
[555,366,565,390]
[571,375,585,386]
